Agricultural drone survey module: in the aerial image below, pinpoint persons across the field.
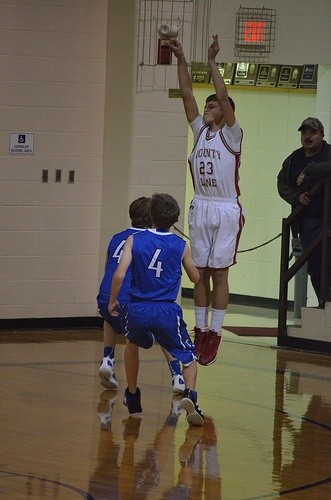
[162,34,246,366]
[83,386,224,499]
[276,117,331,310]
[108,191,206,428]
[95,194,190,393]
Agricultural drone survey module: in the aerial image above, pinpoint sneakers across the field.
[181,389,204,426]
[122,386,143,414]
[170,375,186,393]
[192,326,209,361]
[198,330,221,366]
[98,356,119,388]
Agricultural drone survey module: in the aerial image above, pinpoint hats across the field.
[297,117,323,132]
